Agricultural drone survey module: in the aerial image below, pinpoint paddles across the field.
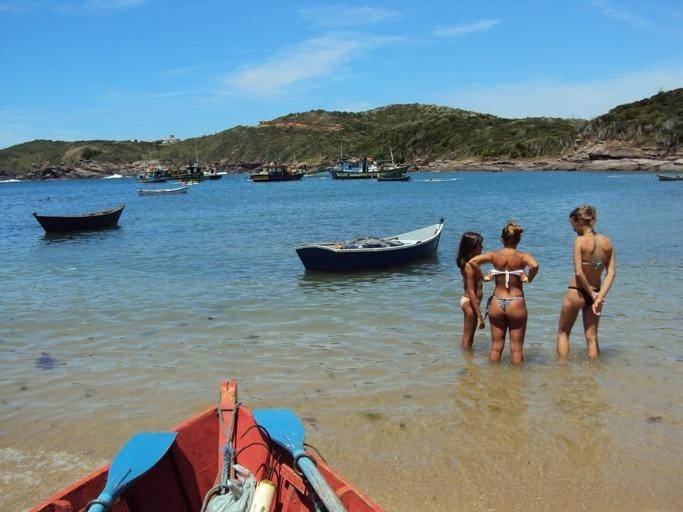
[251,408,348,512]
[89,432,177,511]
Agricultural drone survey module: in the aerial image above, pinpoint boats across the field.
[327,141,412,179]
[655,171,683,182]
[30,203,127,236]
[248,148,305,182]
[137,186,191,197]
[293,217,445,275]
[22,377,390,512]
[135,138,228,183]
[376,174,411,182]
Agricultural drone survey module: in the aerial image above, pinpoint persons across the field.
[456,232,483,349]
[556,205,615,358]
[469,222,539,365]
